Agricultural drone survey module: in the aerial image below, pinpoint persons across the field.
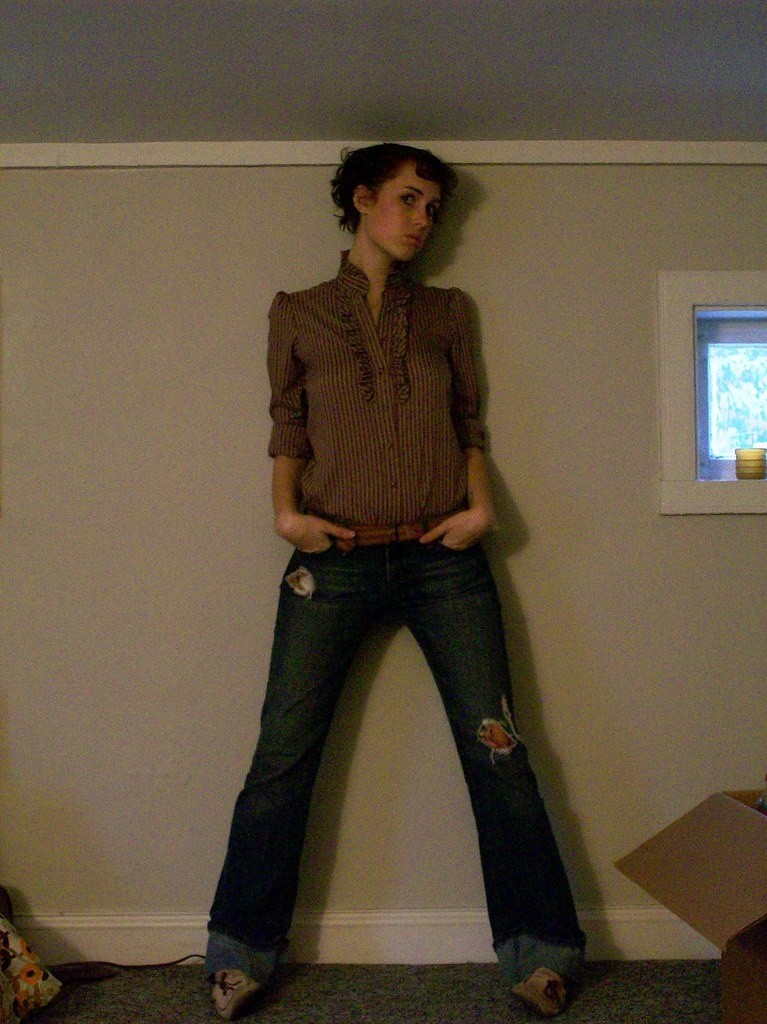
[202,141,592,1019]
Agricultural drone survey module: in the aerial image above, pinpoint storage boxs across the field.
[612,788,767,1024]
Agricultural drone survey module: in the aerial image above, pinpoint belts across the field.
[328,508,467,546]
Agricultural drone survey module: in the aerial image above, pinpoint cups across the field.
[735,448,767,479]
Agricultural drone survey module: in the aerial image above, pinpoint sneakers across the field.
[511,966,565,1017]
[209,967,264,1020]
